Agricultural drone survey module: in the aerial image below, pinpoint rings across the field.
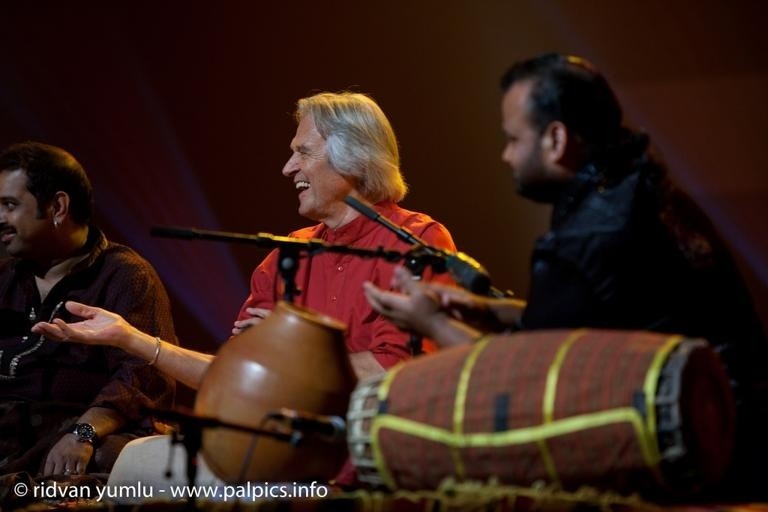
[66,468,77,474]
[388,309,394,319]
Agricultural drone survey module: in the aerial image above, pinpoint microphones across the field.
[278,407,348,444]
[445,255,493,296]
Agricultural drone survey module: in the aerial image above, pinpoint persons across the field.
[360,51,767,460]
[0,141,180,510]
[31,89,461,510]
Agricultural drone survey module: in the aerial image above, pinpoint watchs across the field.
[69,421,103,448]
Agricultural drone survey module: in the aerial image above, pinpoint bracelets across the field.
[144,337,161,367]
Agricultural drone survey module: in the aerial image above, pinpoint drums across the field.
[346,329,732,489]
